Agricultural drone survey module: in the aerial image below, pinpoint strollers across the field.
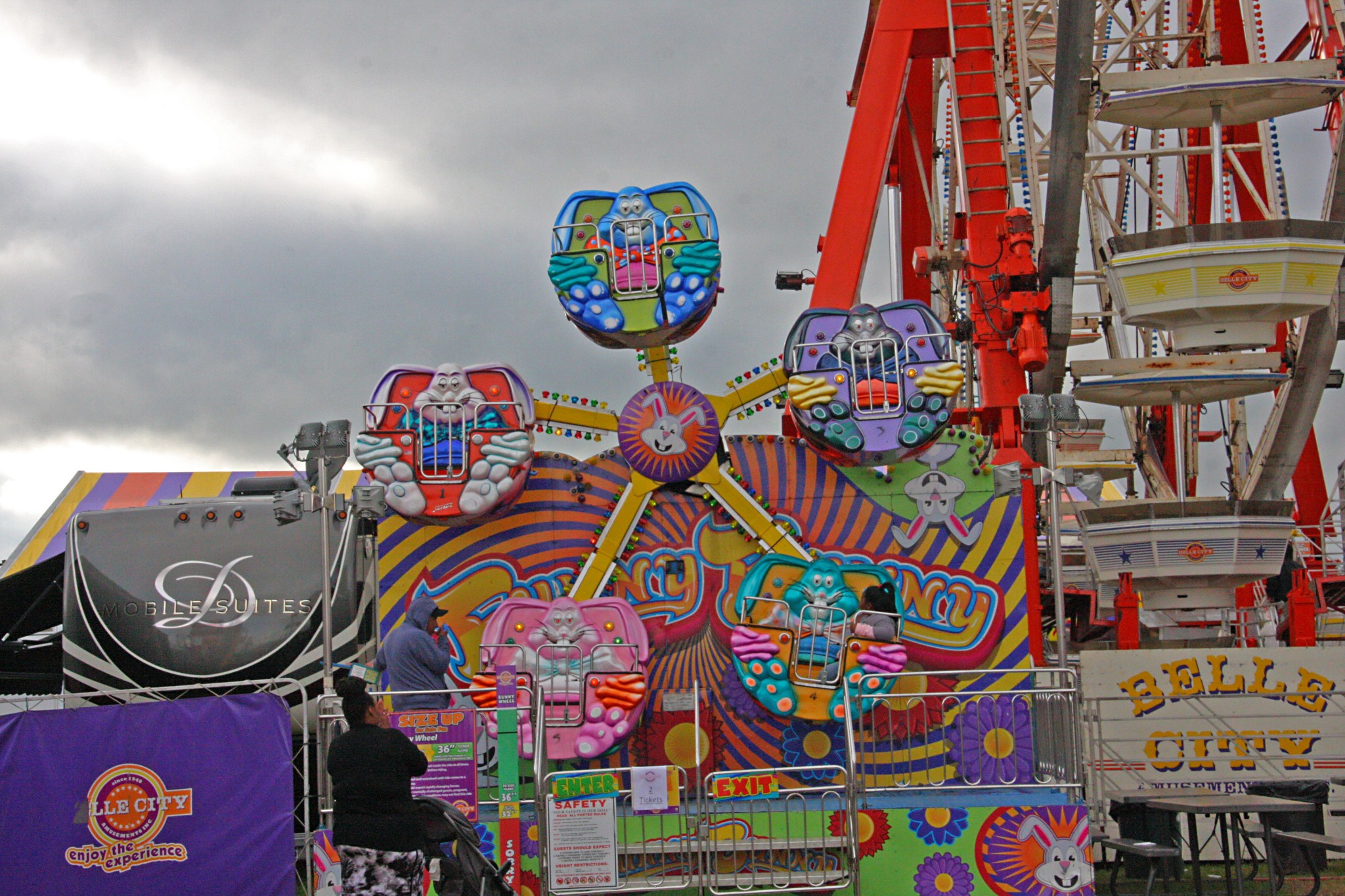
[413,795,520,896]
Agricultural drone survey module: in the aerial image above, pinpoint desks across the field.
[1104,786,1229,896]
[1149,796,1315,896]
[1227,820,1345,896]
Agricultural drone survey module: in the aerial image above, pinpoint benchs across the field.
[1090,825,1179,896]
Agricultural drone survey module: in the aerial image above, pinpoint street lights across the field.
[1019,394,1090,804]
[274,419,389,714]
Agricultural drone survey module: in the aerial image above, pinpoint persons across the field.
[327,678,429,896]
[850,583,898,640]
[372,596,449,709]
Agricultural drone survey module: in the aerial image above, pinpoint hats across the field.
[431,607,448,618]
